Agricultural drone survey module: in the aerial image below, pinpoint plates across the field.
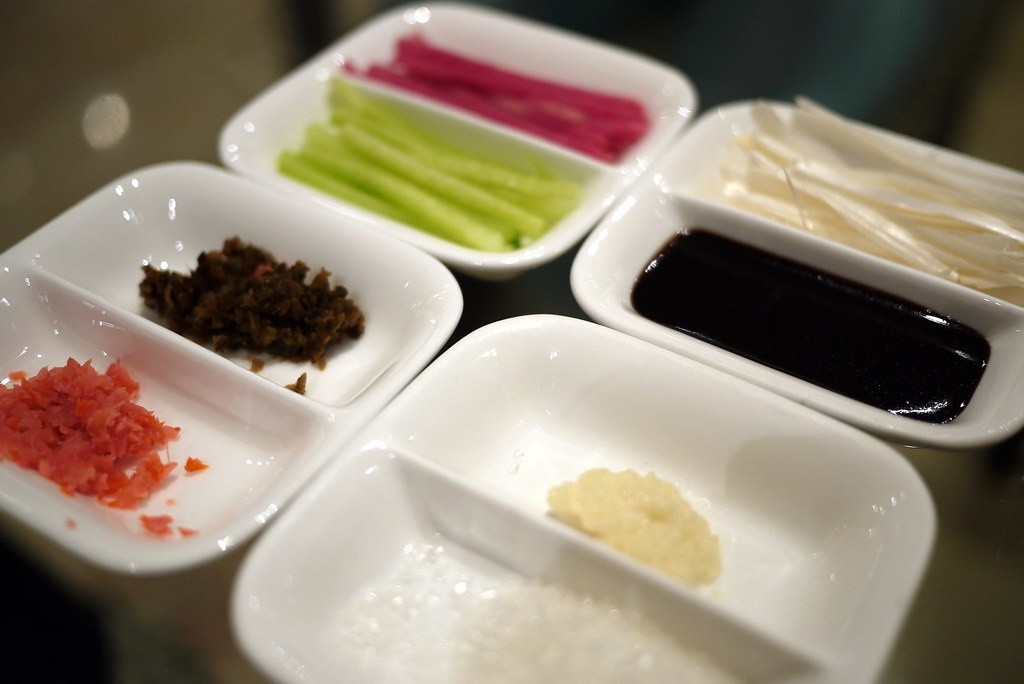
[569,100,1024,448]
[229,315,935,684]
[219,0,698,279]
[0,158,462,622]
